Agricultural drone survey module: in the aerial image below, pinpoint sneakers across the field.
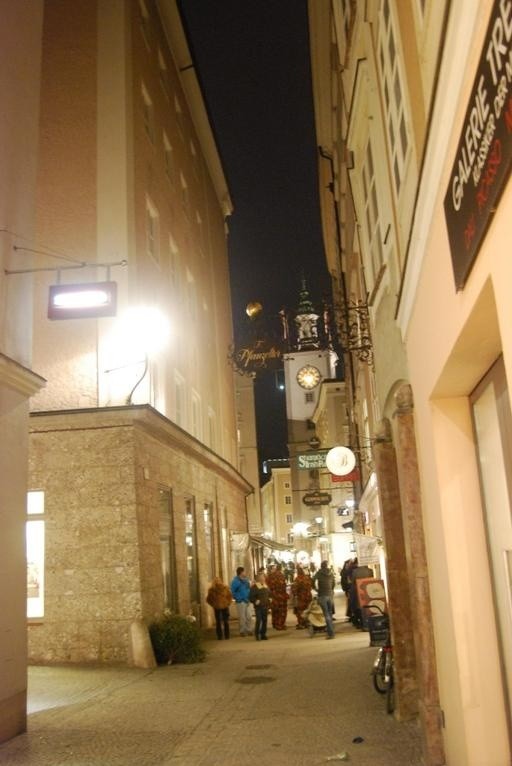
[239,631,267,640]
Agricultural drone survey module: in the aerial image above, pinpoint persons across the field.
[230,566,253,637]
[249,555,358,640]
[206,576,232,640]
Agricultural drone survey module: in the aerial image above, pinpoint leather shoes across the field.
[225,635,229,639]
[218,635,222,639]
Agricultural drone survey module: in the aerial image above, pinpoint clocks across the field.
[296,365,321,390]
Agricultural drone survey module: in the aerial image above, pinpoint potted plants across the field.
[150,612,210,665]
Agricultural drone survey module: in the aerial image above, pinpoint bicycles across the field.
[362,605,394,714]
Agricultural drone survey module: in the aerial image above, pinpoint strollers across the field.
[302,596,329,638]
